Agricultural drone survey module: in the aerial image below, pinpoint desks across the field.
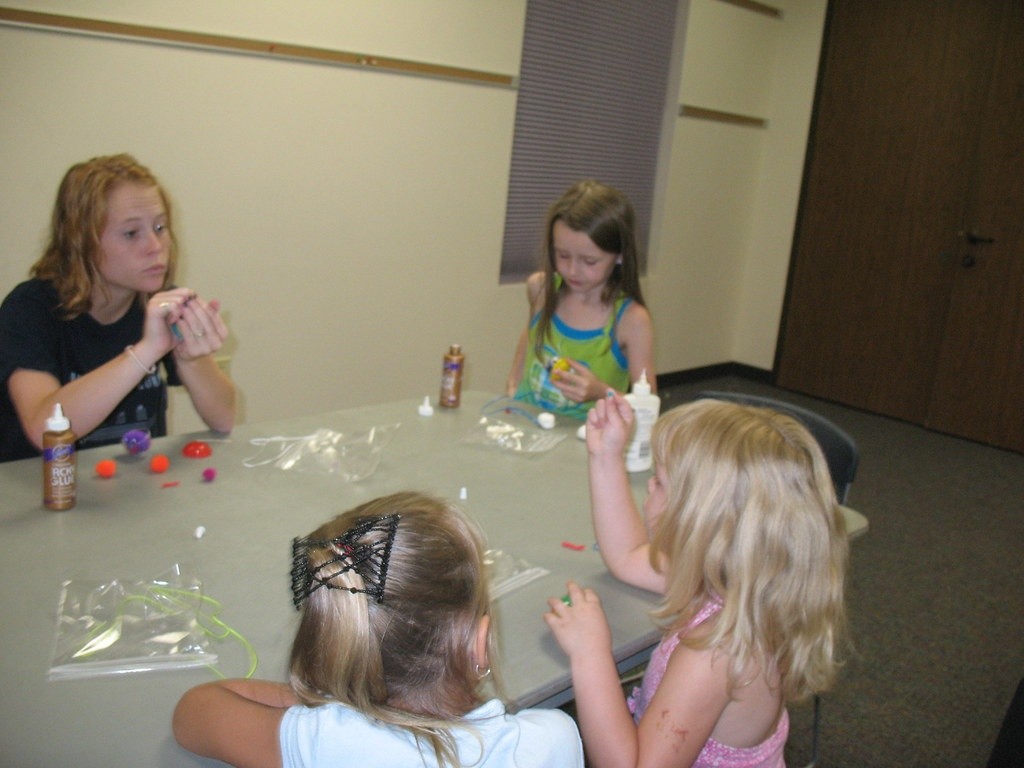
[0,391,691,768]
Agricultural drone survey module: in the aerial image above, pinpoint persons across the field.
[543,388,852,768]
[172,490,586,768]
[506,182,659,427]
[1,154,236,452]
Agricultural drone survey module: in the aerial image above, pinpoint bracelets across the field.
[126,345,156,377]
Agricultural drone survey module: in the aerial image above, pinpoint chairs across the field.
[657,388,857,766]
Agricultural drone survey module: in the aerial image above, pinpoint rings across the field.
[195,331,206,339]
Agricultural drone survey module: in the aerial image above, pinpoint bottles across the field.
[623,369,660,473]
[440,345,464,408]
[43,403,76,512]
